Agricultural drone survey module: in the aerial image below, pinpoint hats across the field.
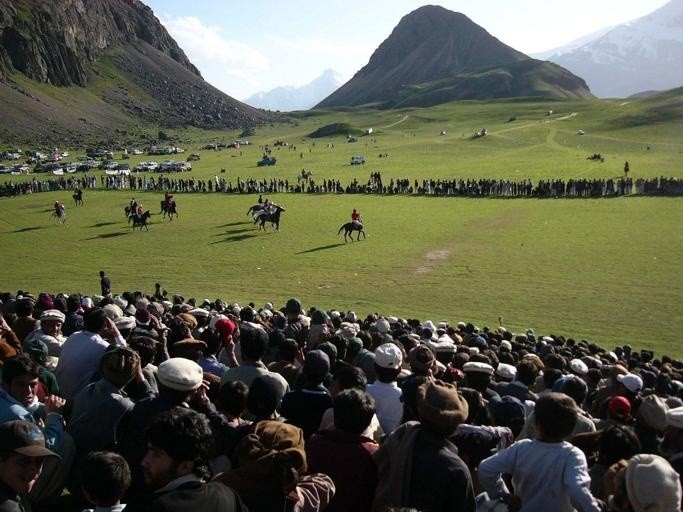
[435,334,517,380]
[496,396,526,427]
[607,395,683,429]
[375,343,403,369]
[569,359,589,375]
[616,373,643,393]
[99,295,229,391]
[331,310,399,340]
[1,420,60,459]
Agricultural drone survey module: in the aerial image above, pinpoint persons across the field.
[99,271,111,296]
[99,172,479,198]
[258,194,269,212]
[479,161,682,198]
[352,209,361,225]
[2,175,96,197]
[3,283,683,511]
[131,191,173,213]
[55,201,60,211]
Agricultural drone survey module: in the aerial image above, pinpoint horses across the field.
[72,189,83,207]
[128,210,151,231]
[298,172,312,183]
[337,219,367,243]
[157,201,179,221]
[246,203,287,233]
[124,202,138,218]
[49,205,67,226]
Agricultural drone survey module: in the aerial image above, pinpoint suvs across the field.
[577,130,584,135]
